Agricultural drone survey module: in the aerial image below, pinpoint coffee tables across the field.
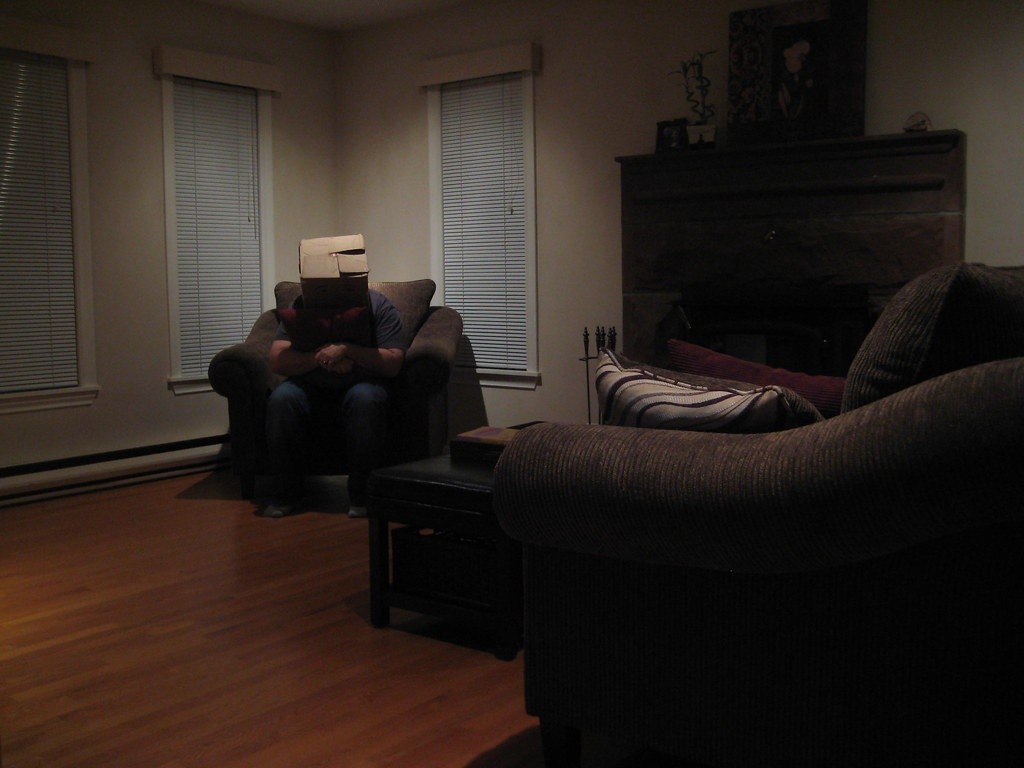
[367,421,547,661]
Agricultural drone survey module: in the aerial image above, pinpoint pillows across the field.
[272,304,372,387]
[842,261,1024,416]
[668,338,844,422]
[597,347,825,433]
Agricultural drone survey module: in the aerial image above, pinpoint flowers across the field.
[668,48,718,124]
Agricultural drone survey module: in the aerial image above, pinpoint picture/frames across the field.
[655,117,688,153]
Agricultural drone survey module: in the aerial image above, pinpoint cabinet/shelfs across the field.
[615,129,967,377]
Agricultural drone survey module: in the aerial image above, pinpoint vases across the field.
[686,125,716,149]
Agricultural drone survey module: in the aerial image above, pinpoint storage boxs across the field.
[450,422,545,469]
[392,526,499,607]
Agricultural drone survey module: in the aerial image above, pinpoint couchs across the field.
[495,356,1024,768]
[208,278,463,498]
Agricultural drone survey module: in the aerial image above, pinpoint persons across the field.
[257,235,406,517]
